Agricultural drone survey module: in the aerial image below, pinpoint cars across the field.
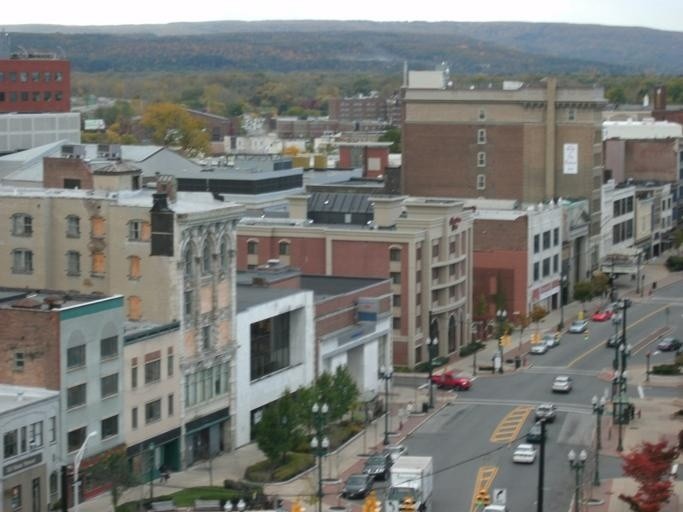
[527,296,636,354]
[341,443,406,512]
[656,335,682,352]
[525,423,547,444]
[508,443,538,465]
[481,503,507,512]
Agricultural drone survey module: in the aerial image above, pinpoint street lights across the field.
[422,335,441,411]
[71,429,100,512]
[308,435,332,512]
[221,498,247,512]
[496,305,511,376]
[568,336,634,512]
[378,360,397,445]
[311,392,331,443]
[558,272,568,328]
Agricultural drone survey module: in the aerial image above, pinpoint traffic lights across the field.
[361,496,371,512]
[471,488,491,507]
[400,497,415,512]
[372,496,380,512]
[499,336,506,347]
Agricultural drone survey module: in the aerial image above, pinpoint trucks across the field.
[386,453,436,512]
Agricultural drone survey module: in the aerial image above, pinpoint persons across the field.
[158,464,169,485]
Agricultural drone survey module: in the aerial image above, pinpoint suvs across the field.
[551,373,574,393]
[534,402,557,422]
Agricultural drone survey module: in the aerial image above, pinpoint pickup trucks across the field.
[428,368,473,392]
